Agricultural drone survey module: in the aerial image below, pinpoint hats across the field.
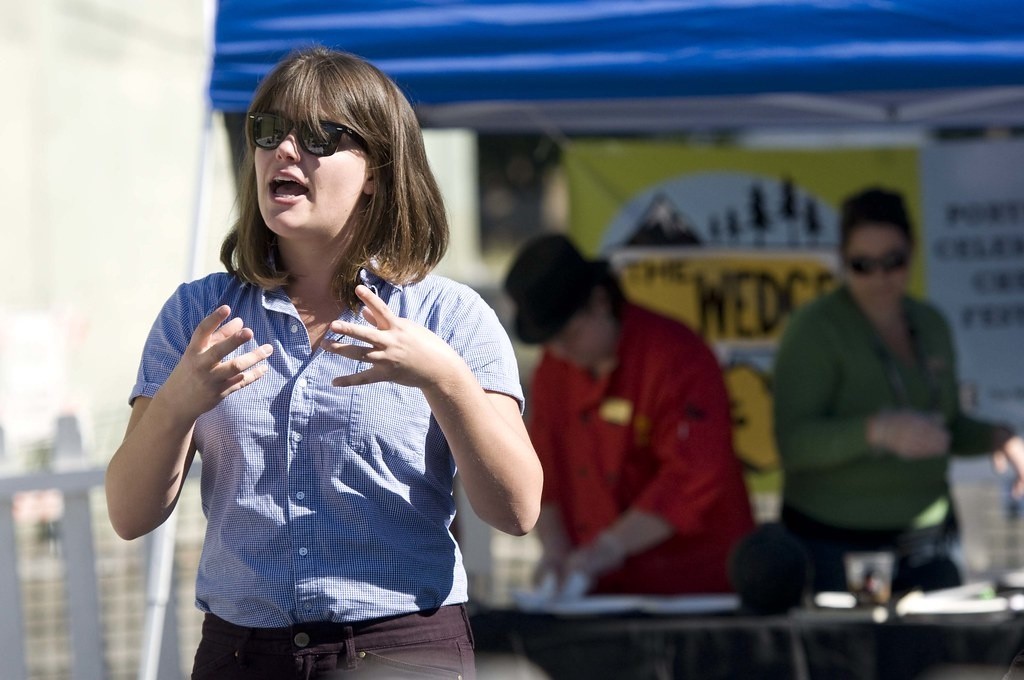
[503,229,612,346]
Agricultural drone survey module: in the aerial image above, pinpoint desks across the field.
[464,608,1024,680]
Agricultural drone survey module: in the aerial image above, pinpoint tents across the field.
[134,0,1024,680]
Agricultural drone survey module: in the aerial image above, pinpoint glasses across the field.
[249,111,374,159]
[846,247,912,277]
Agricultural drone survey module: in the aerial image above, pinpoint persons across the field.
[776,189,1024,680]
[505,233,758,680]
[105,53,544,679]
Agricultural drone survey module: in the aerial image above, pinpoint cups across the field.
[842,549,895,606]
